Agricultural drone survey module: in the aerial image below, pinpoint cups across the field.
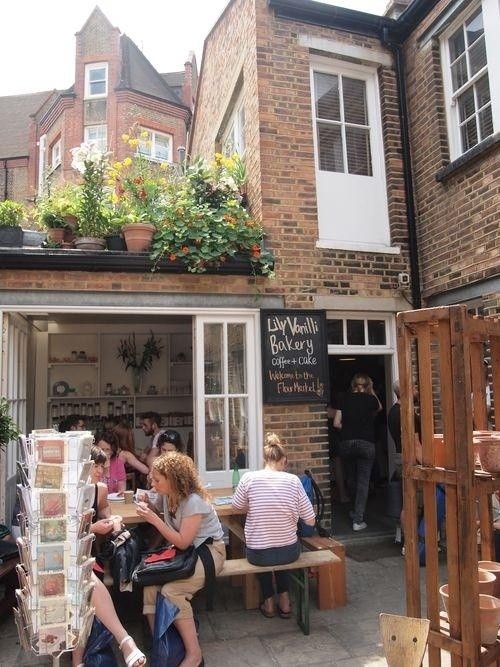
[124,491,134,505]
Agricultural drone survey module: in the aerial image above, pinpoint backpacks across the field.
[294,471,331,538]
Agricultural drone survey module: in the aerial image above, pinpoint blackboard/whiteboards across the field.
[260,309,330,405]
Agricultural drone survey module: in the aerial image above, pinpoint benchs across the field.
[300,534,347,609]
[216,550,342,635]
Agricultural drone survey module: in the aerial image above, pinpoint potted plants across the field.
[31,200,81,248]
[115,328,164,394]
[146,150,276,303]
[38,208,68,246]
[0,199,29,247]
[59,182,83,248]
[21,213,47,245]
[99,192,137,251]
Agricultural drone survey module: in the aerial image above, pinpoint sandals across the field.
[259,601,278,617]
[277,600,293,619]
[119,636,147,667]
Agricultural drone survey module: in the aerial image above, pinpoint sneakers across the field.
[352,522,367,531]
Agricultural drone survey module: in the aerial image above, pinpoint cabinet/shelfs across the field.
[35,332,193,463]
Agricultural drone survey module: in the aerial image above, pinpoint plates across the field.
[106,491,125,502]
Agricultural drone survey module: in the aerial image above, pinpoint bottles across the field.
[80,402,87,416]
[70,351,77,362]
[66,402,72,416]
[74,404,80,416]
[232,465,240,493]
[88,405,93,416]
[94,403,100,416]
[108,401,113,417]
[59,404,64,416]
[128,405,134,428]
[77,351,87,363]
[51,404,58,419]
[116,407,121,417]
[121,401,127,415]
[105,383,113,395]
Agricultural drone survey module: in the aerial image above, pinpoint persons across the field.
[138,432,184,490]
[333,375,383,531]
[141,411,165,456]
[72,446,147,667]
[94,430,126,496]
[387,379,422,558]
[115,424,150,477]
[135,451,225,667]
[59,415,85,434]
[231,433,316,618]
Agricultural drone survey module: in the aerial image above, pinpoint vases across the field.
[48,228,64,246]
[121,224,157,252]
[1,224,22,246]
[23,231,46,246]
[103,234,125,251]
[72,237,107,250]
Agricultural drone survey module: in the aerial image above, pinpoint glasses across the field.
[160,434,177,440]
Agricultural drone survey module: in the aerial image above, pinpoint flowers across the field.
[106,103,169,224]
[70,138,114,251]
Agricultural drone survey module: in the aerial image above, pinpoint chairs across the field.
[379,613,431,667]
